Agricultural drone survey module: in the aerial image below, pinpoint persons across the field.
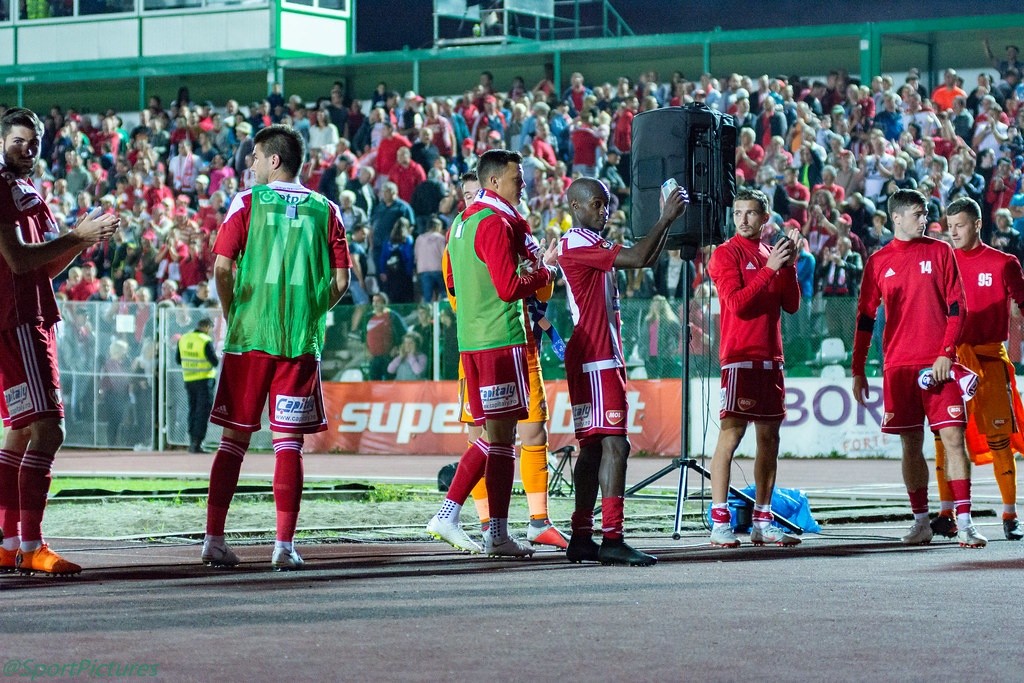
[674,37,1024,378]
[923,196,1024,541]
[28,85,285,447]
[849,188,987,549]
[480,70,729,381]
[176,318,220,453]
[425,149,559,560]
[280,81,485,381]
[706,190,804,548]
[199,122,353,573]
[440,173,572,550]
[556,175,689,568]
[0,107,120,582]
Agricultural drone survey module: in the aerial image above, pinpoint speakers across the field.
[629,101,736,251]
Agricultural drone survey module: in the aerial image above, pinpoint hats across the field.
[41,73,1024,289]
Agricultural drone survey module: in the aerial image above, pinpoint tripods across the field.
[593,245,805,542]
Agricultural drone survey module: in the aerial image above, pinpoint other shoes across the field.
[189,440,210,454]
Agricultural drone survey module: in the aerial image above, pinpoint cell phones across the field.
[662,178,679,203]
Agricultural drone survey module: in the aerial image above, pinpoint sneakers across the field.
[271,547,305,571]
[426,515,482,555]
[597,539,658,566]
[15,543,82,577]
[0,546,20,573]
[566,537,600,563]
[201,539,242,567]
[751,525,802,547]
[485,532,537,558]
[527,522,571,549]
[1003,519,1024,541]
[930,514,958,539]
[710,524,742,548]
[901,524,933,545]
[957,525,987,548]
